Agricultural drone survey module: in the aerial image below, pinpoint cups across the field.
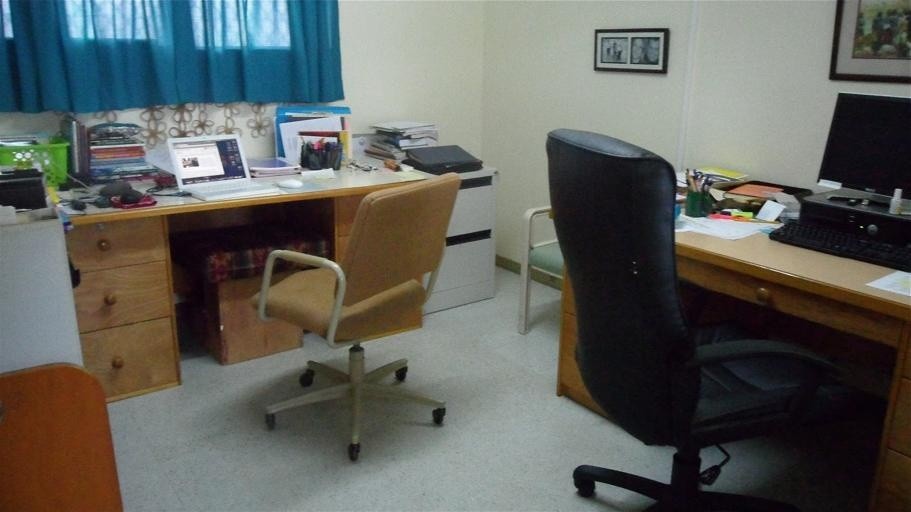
[686,190,712,218]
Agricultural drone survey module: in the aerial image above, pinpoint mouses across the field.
[277,179,304,189]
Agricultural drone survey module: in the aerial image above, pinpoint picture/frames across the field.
[594,28,668,72]
[829,0,911,83]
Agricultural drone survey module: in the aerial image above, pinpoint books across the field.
[675,164,753,196]
[364,121,441,163]
[724,183,785,207]
[273,104,355,168]
[86,122,157,183]
[248,158,304,179]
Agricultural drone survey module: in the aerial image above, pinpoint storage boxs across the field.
[185,268,303,365]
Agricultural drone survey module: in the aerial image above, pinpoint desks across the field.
[556,187,911,512]
[47,159,446,406]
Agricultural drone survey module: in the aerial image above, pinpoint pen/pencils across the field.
[686,168,713,193]
[306,139,342,170]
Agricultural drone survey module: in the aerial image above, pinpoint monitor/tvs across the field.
[817,92,911,206]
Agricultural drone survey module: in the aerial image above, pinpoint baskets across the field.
[0,137,71,186]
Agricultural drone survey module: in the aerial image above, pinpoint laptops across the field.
[168,133,280,201]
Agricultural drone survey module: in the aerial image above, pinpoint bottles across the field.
[889,188,903,215]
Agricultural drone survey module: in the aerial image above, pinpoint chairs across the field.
[518,206,564,334]
[248,171,462,461]
[544,128,860,512]
[0,360,123,512]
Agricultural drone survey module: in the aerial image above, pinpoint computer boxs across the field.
[800,188,911,245]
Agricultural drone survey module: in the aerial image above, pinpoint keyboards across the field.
[770,222,911,273]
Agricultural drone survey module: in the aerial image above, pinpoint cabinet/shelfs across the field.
[420,172,496,315]
